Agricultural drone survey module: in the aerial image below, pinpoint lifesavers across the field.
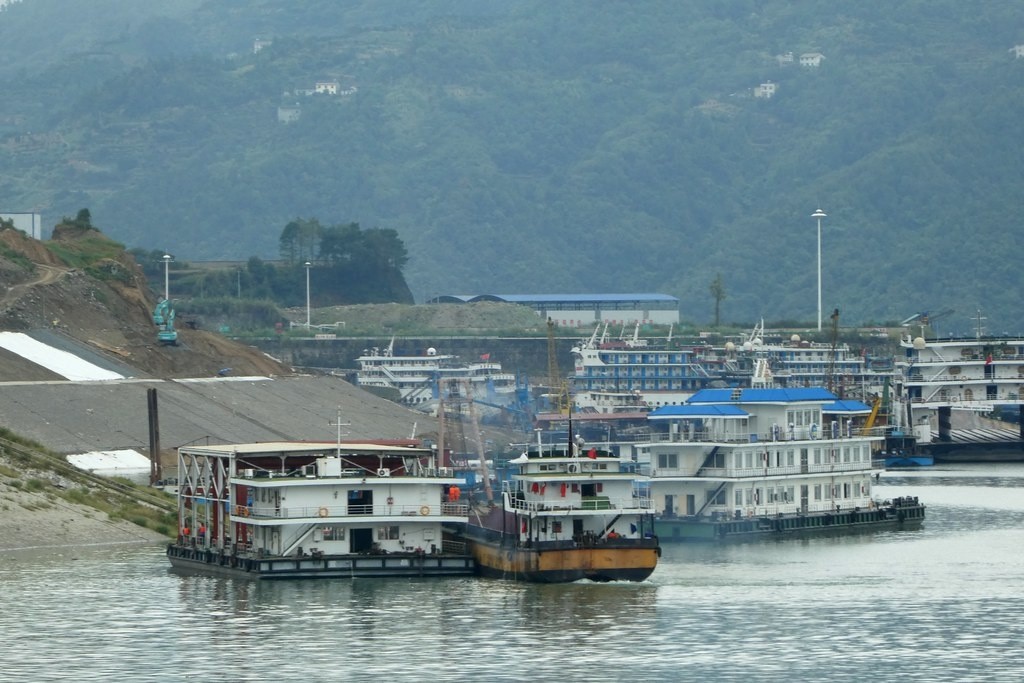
[204,548,252,573]
[879,509,884,519]
[851,511,857,521]
[800,516,806,526]
[420,505,430,515]
[507,552,512,561]
[777,519,785,532]
[319,507,328,517]
[235,506,249,517]
[962,376,966,380]
[952,397,957,401]
[826,514,832,524]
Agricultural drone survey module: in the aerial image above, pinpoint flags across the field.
[986,354,993,365]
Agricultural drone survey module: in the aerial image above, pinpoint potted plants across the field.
[572,534,578,548]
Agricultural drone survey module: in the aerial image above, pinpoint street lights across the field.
[811,209,829,331]
[304,261,311,329]
[163,255,170,299]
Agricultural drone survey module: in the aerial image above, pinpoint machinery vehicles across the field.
[154,299,179,346]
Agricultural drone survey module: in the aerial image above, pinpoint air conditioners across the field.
[951,396,960,401]
[378,469,390,476]
[566,464,580,473]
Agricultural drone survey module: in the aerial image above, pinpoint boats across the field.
[165,406,481,580]
[355,347,518,411]
[644,384,927,542]
[432,404,665,585]
[881,432,935,466]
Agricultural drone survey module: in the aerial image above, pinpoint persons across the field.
[183,523,191,547]
[198,522,205,537]
[448,485,461,514]
